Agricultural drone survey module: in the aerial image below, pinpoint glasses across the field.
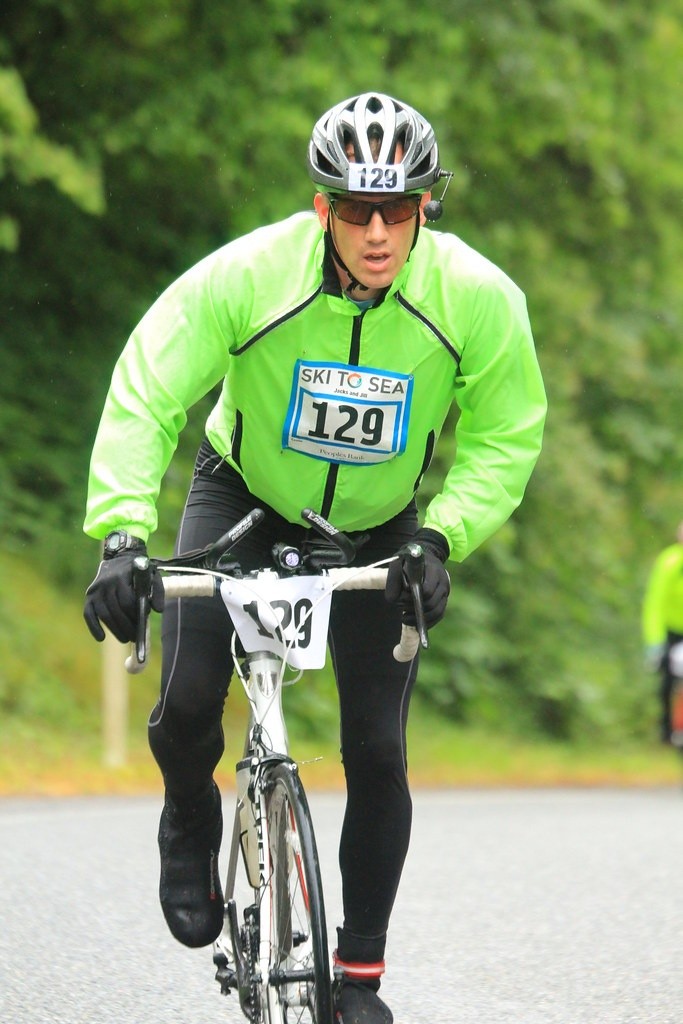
[323,191,422,226]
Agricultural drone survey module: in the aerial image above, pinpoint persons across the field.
[640,519,683,749]
[80,90,550,1024]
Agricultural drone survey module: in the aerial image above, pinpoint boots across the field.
[329,927,394,1024]
[158,777,222,949]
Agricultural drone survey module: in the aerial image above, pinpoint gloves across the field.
[384,527,451,632]
[83,548,166,644]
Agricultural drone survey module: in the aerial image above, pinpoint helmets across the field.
[305,91,440,195]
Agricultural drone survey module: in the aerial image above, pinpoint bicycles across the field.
[122,509,434,1024]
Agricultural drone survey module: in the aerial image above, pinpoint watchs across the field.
[103,529,146,559]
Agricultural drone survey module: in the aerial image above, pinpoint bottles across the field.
[236,755,260,889]
